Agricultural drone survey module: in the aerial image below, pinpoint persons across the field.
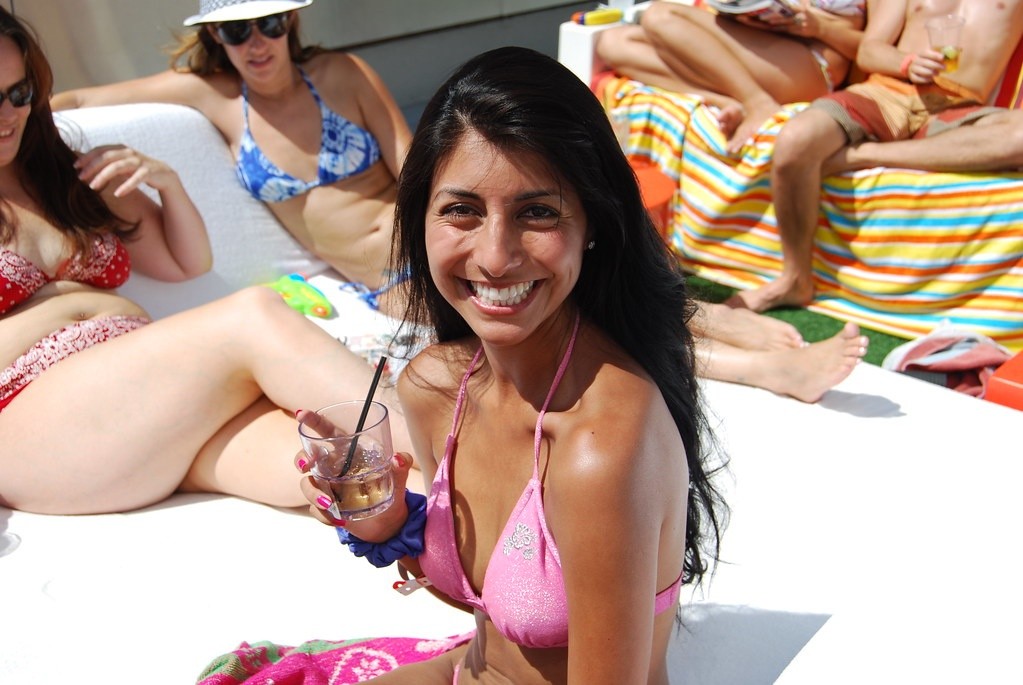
[0,7,413,513]
[594,1,863,156]
[50,0,869,405]
[719,1,1023,317]
[212,42,713,685]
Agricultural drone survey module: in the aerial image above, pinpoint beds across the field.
[559,10,1023,356]
[1,101,1022,685]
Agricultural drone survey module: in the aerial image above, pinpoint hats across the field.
[183,0,313,27]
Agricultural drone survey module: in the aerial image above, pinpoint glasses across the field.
[0,78,34,108]
[211,13,288,46]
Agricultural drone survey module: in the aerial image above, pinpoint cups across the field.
[297,400,397,522]
[925,14,966,74]
